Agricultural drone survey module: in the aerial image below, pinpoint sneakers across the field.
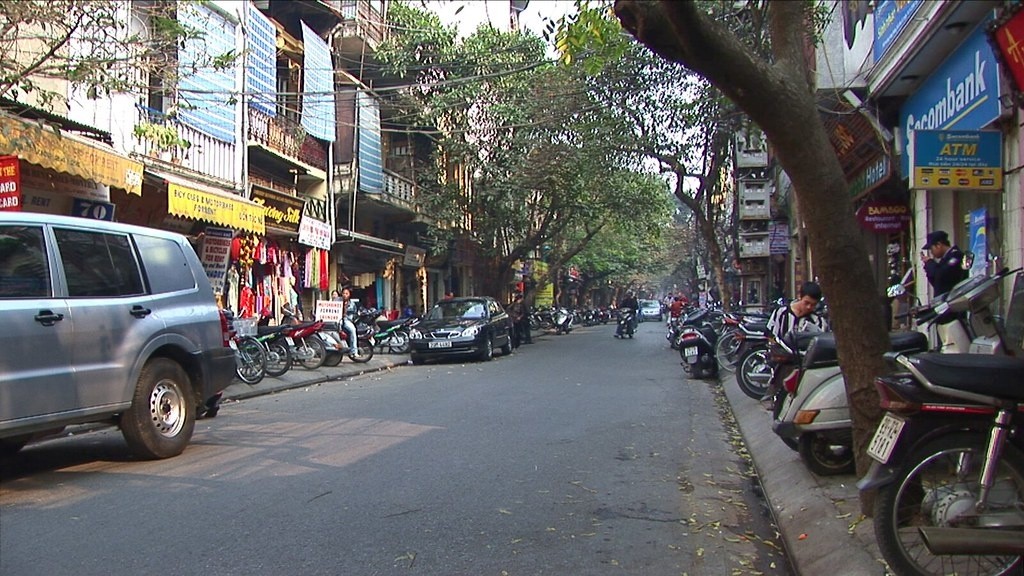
[350,353,362,358]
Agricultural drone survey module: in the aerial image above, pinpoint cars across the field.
[408,295,513,366]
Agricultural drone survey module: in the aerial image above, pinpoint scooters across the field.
[510,299,619,334]
[665,261,1010,476]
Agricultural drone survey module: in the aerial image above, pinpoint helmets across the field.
[626,289,632,296]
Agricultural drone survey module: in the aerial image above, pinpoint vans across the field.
[640,300,663,322]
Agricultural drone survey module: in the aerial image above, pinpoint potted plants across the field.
[166,127,189,167]
[133,121,165,159]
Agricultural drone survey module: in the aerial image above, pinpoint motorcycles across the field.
[857,345,1024,576]
[614,303,638,338]
[220,302,419,384]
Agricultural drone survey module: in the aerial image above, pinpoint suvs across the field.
[0,211,239,463]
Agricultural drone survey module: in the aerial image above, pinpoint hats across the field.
[921,231,948,250]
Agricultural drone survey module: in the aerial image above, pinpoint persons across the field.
[662,292,676,322]
[920,231,970,351]
[764,280,829,421]
[328,287,362,358]
[506,295,524,352]
[671,297,685,317]
[613,290,639,338]
[444,289,454,299]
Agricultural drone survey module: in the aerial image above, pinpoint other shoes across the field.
[614,333,620,337]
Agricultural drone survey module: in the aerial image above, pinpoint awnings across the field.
[1,111,144,196]
[143,168,266,238]
